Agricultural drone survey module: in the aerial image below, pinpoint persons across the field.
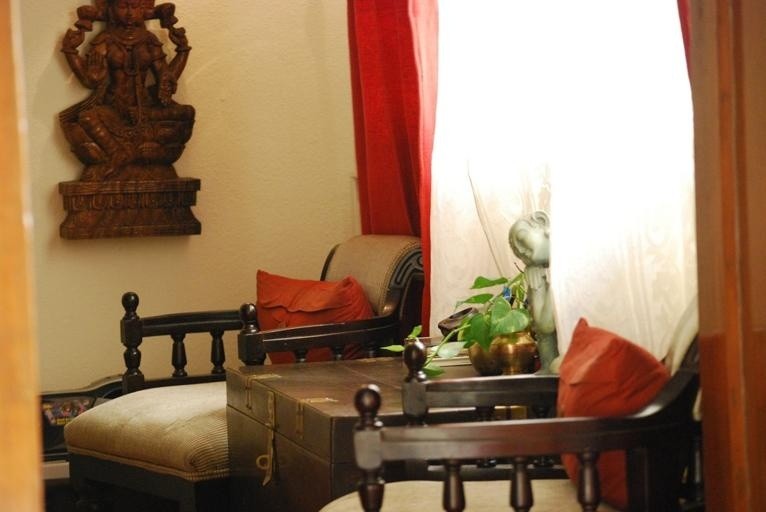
[58,0,196,180]
[508,207,559,374]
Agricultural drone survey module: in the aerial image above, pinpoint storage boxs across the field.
[216,354,528,512]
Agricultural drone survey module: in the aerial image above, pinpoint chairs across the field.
[308,289,711,510]
[60,232,418,512]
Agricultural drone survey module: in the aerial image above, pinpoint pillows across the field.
[553,312,689,512]
[251,265,396,366]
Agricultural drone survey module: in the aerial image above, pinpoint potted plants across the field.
[373,268,538,375]
